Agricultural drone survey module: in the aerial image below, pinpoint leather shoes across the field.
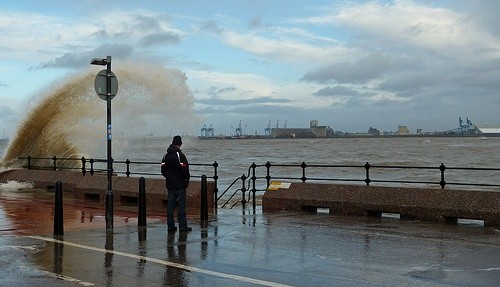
[179,226,192,232]
[168,226,177,231]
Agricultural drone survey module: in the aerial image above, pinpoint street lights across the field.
[90,55,115,198]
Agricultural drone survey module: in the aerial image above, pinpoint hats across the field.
[172,135,182,146]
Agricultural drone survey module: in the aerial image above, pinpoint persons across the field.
[161,136,194,233]
